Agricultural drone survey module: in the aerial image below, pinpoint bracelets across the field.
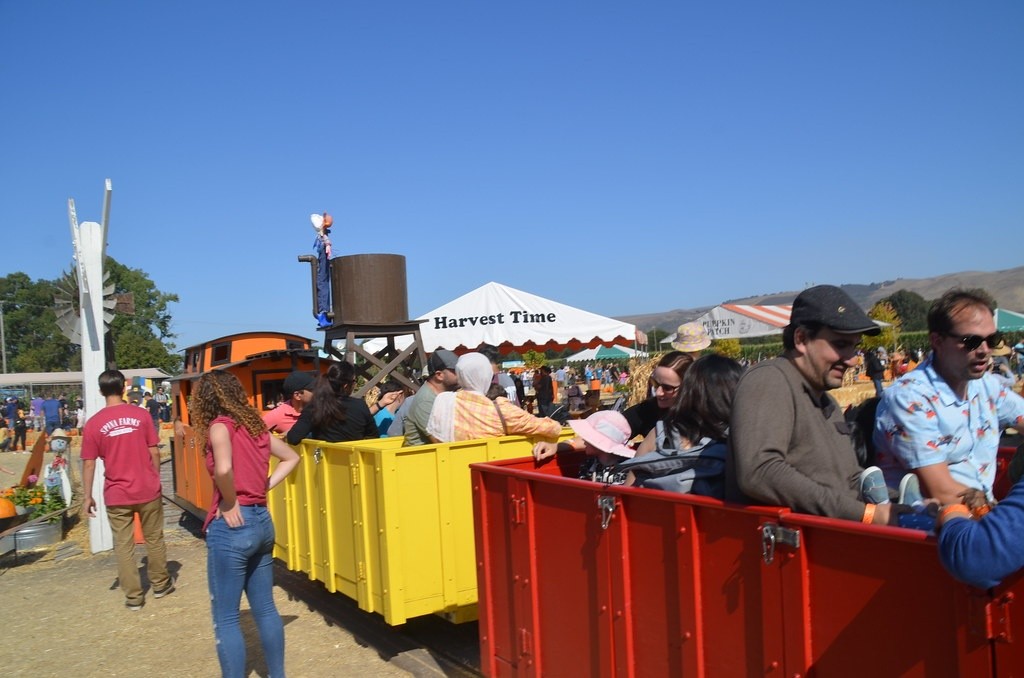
[862,503,876,525]
[374,402,384,410]
[937,502,970,524]
[970,502,991,521]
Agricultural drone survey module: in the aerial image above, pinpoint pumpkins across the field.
[159,419,174,429]
[0,488,42,519]
[10,429,77,452]
[602,386,614,393]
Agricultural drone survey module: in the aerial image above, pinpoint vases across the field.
[13,515,65,550]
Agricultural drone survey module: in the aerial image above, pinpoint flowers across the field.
[8,457,70,528]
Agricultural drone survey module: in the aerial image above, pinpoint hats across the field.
[877,347,886,353]
[282,371,318,397]
[790,286,884,337]
[427,350,459,374]
[566,411,639,461]
[48,428,72,443]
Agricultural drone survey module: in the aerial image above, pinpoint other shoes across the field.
[859,466,890,505]
[897,473,922,505]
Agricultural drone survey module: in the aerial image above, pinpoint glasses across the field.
[649,376,681,391]
[943,331,1004,351]
[352,378,358,383]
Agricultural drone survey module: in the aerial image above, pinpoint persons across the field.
[853,332,1023,397]
[0,384,169,455]
[374,354,746,506]
[729,285,914,526]
[871,288,1024,505]
[287,362,406,445]
[859,443,1023,589]
[387,393,416,437]
[186,370,301,678]
[401,348,459,446]
[261,362,404,444]
[84,370,175,610]
[564,407,632,485]
[372,380,405,438]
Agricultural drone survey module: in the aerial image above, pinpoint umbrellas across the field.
[564,343,650,362]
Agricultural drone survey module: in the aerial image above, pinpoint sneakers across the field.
[152,577,175,599]
[126,601,143,610]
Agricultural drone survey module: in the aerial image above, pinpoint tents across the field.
[364,281,648,369]
[658,302,892,346]
